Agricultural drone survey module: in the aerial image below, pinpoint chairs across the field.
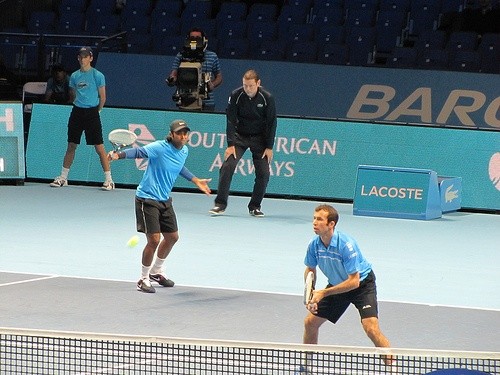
[0,0,500,98]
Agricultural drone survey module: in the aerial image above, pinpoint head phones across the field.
[203,36,209,45]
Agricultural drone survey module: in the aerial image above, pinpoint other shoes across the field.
[296,368,311,375]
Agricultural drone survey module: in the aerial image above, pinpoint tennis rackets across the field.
[107,129,136,164]
[303,272,316,309]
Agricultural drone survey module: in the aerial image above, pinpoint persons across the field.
[209,69,277,217]
[294,205,401,375]
[50,46,114,190]
[44,64,76,106]
[165,28,222,113]
[107,120,211,293]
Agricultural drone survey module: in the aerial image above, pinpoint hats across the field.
[78,48,93,56]
[170,120,190,132]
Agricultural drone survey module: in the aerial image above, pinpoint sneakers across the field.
[250,209,264,216]
[102,182,115,190]
[149,272,174,287]
[137,279,155,293]
[209,206,224,214]
[50,177,67,186]
